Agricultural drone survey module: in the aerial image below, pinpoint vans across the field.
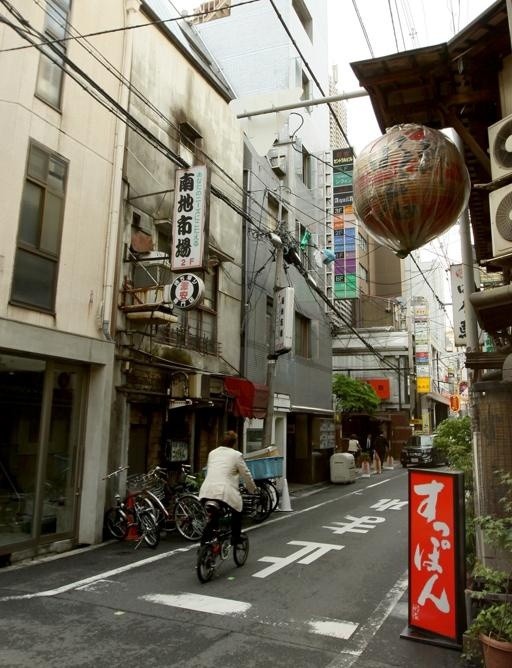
[400,434,450,466]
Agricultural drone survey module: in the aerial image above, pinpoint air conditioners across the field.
[488,183,511,258]
[489,112,511,183]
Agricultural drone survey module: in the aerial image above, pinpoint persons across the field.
[347,434,361,458]
[373,430,389,470]
[199,430,258,546]
[362,433,372,453]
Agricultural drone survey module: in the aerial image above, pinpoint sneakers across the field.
[231,536,248,547]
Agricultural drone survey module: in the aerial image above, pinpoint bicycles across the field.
[99,462,279,548]
[197,485,262,583]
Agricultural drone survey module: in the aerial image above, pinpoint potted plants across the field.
[460,600,512,668]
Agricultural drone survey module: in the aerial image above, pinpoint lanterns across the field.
[354,124,468,260]
